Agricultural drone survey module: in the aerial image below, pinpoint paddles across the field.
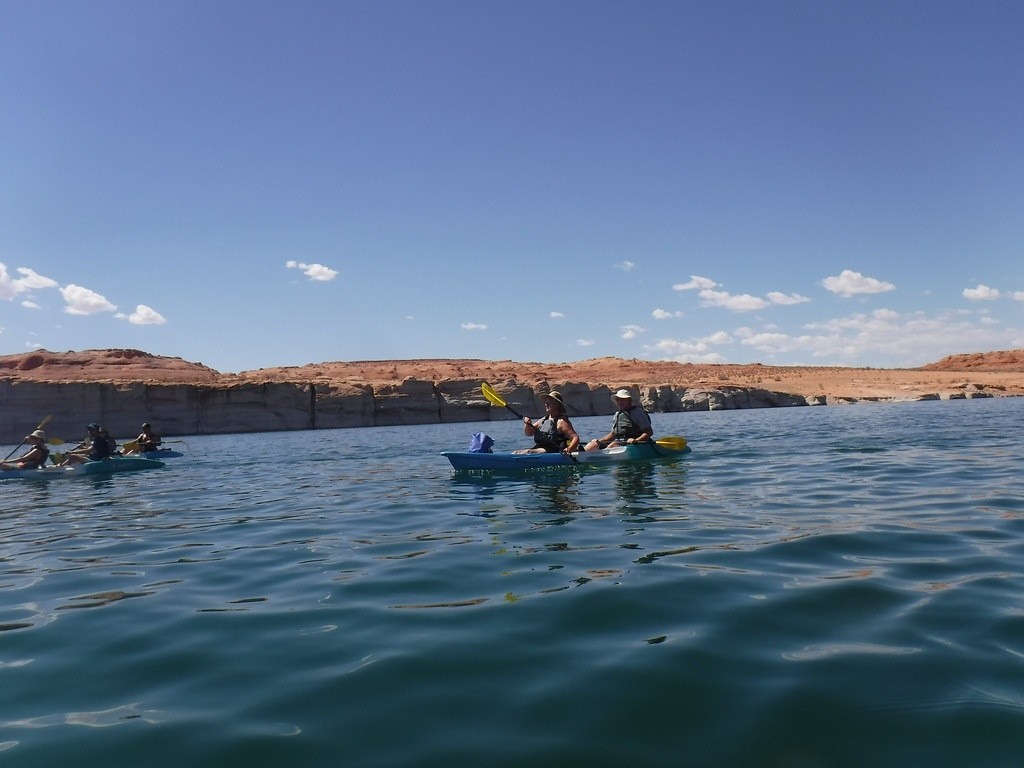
[4,415,52,460]
[48,438,143,450]
[579,436,687,450]
[481,382,582,465]
[134,440,182,444]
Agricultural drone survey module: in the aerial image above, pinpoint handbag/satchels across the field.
[468,432,496,453]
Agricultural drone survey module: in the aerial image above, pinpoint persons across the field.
[576,389,653,452]
[40,423,109,468]
[0,430,45,471]
[117,422,157,457]
[82,428,113,455]
[512,391,579,456]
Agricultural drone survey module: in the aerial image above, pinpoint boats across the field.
[49,448,186,465]
[0,453,165,480]
[438,436,689,471]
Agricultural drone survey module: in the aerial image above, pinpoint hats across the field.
[30,430,46,442]
[539,391,566,413]
[85,423,98,431]
[611,390,632,405]
[141,422,150,429]
[99,427,108,433]
